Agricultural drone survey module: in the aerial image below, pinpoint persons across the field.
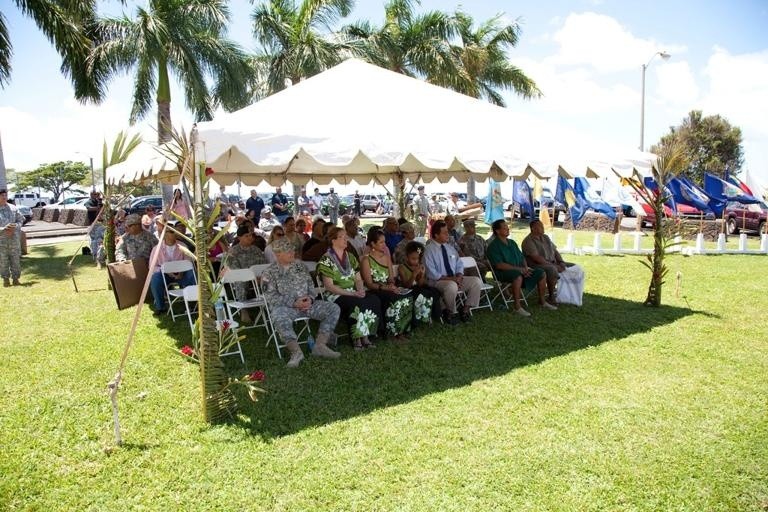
[412,186,433,237]
[354,190,361,218]
[207,189,340,371]
[271,185,291,226]
[318,217,582,351]
[0,187,27,288]
[311,187,324,216]
[85,190,194,313]
[326,187,340,225]
[6,197,27,255]
[213,185,229,205]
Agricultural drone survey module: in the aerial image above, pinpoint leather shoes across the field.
[152,310,168,318]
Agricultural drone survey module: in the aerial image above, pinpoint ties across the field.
[441,244,454,277]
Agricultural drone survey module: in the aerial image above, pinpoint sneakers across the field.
[538,301,558,311]
[446,313,474,326]
[3,278,10,287]
[13,278,21,285]
[513,308,531,318]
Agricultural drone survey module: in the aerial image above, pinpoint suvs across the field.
[724,193,768,238]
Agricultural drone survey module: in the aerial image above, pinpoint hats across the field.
[385,217,398,225]
[463,219,478,227]
[418,185,425,190]
[260,208,271,214]
[125,213,142,226]
[272,239,296,253]
[400,222,415,232]
[429,191,458,197]
[0,189,7,194]
[237,226,251,236]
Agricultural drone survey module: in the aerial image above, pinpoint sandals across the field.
[354,343,365,352]
[362,342,378,348]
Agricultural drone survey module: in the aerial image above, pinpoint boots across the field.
[286,339,305,368]
[310,330,343,360]
[240,308,252,323]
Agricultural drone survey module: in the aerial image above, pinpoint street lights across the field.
[74,151,95,191]
[640,48,675,151]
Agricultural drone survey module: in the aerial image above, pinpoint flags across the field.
[556,174,590,231]
[601,165,768,221]
[531,175,555,235]
[512,175,535,222]
[569,176,616,230]
[482,179,504,229]
